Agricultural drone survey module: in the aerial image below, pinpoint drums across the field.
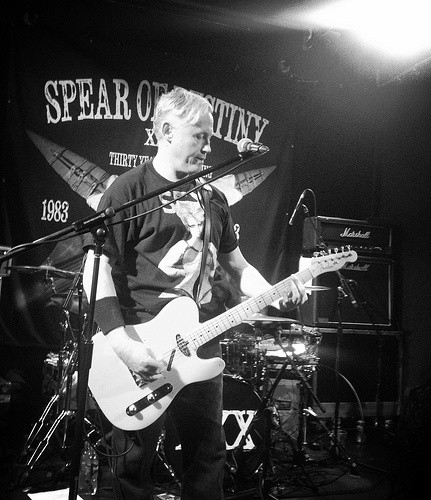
[161,376,268,488]
[217,335,265,375]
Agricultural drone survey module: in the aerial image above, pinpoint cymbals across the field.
[305,284,331,293]
[242,313,299,323]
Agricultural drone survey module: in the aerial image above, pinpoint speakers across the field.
[290,325,403,453]
[299,251,397,330]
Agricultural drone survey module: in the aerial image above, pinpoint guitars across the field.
[88,244,360,432]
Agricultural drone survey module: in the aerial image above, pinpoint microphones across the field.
[289,190,307,226]
[236,138,269,154]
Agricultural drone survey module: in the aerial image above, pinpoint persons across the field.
[82,88,312,499]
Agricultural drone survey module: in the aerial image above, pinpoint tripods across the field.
[9,280,113,492]
